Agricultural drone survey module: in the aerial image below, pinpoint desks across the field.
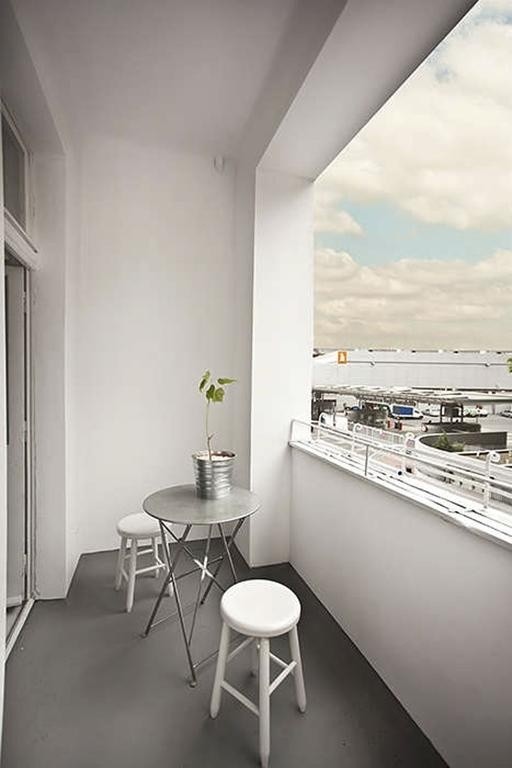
[141,483,262,687]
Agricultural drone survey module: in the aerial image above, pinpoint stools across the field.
[209,579,308,768]
[114,511,173,612]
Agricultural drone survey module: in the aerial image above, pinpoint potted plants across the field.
[191,370,237,500]
